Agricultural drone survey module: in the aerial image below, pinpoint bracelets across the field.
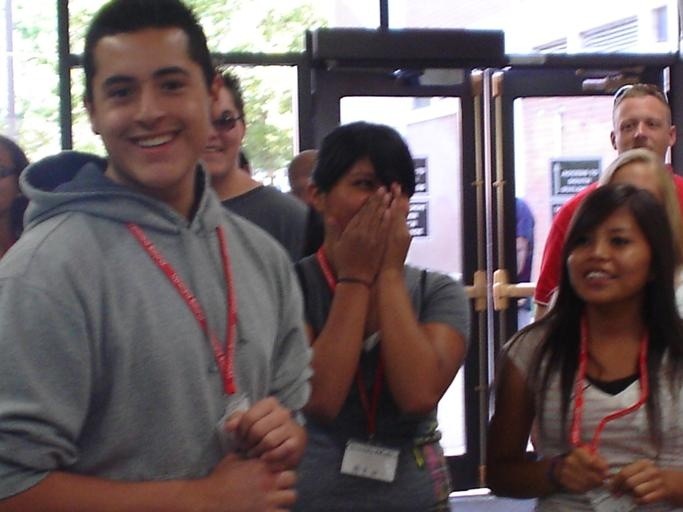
[548,455,558,487]
[335,278,370,287]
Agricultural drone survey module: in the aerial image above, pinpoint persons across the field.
[514,199,536,314]
[1,1,312,512]
[290,122,469,511]
[485,186,682,512]
[1,136,31,255]
[287,151,319,206]
[536,83,682,325]
[602,149,682,324]
[208,68,319,259]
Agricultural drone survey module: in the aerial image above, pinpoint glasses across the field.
[211,114,244,130]
